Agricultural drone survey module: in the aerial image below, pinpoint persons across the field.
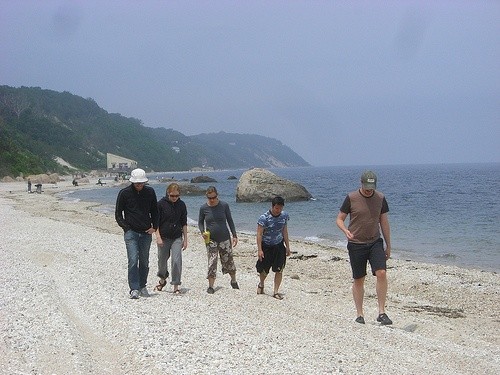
[198,186,239,294]
[115,169,160,300]
[155,174,174,181]
[255,196,291,300]
[69,170,128,186]
[26,178,42,194]
[154,183,188,293]
[335,169,392,324]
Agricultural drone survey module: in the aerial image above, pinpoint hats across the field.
[361,169,377,191]
[129,168,148,183]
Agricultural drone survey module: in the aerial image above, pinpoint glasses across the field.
[207,196,217,199]
[170,195,180,197]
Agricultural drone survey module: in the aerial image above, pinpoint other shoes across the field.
[207,287,214,294]
[355,316,365,324]
[141,287,150,297]
[129,289,139,299]
[230,279,239,289]
[376,313,392,325]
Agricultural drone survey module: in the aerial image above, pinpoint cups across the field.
[203,232,210,243]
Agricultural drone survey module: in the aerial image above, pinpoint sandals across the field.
[174,289,182,296]
[257,282,265,295]
[273,292,284,300]
[153,280,167,291]
